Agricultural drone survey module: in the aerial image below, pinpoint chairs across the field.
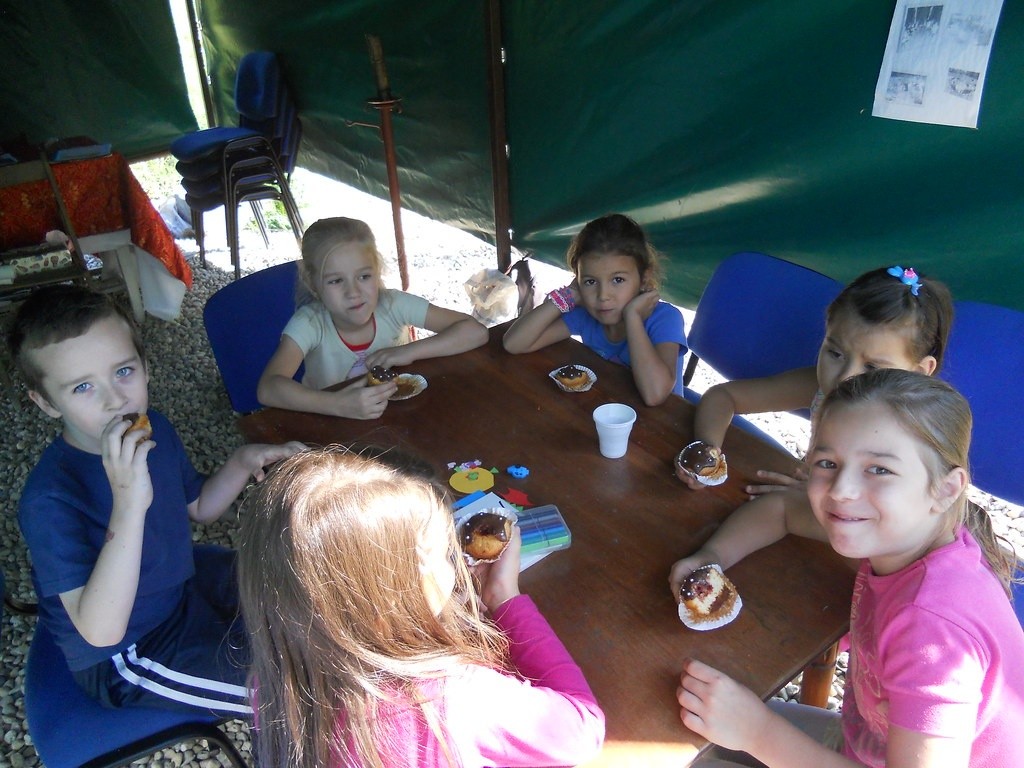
[0,531,257,768]
[926,298,1024,630]
[683,251,849,459]
[201,258,307,415]
[166,46,304,284]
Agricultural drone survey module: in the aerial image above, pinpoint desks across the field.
[0,134,193,329]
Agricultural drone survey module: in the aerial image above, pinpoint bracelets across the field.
[548,287,575,314]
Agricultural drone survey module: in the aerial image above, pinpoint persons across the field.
[504,214,689,407]
[674,264,953,492]
[256,217,490,420]
[8,285,309,721]
[238,448,607,768]
[670,368,1024,768]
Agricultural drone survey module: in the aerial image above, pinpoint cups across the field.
[593,402,637,459]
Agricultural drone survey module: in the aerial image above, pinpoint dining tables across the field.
[236,319,859,768]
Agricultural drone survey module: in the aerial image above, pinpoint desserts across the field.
[460,511,512,560]
[681,442,726,478]
[121,412,153,445]
[681,567,737,623]
[367,365,398,386]
[556,366,590,390]
